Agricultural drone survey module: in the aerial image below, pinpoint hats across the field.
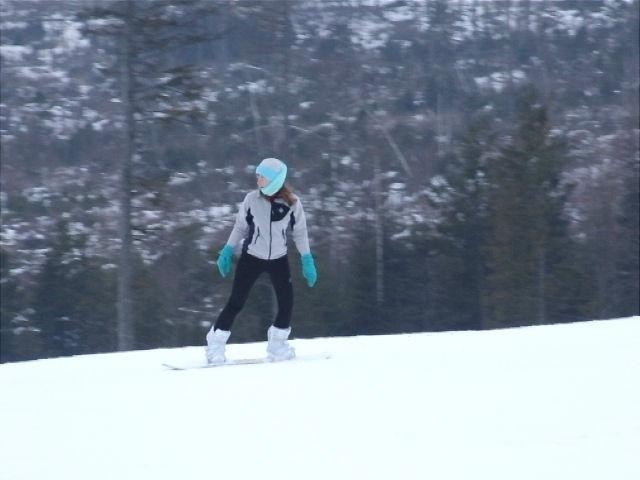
[256,158,283,181]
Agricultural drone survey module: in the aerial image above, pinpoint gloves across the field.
[301,253,317,287]
[217,244,235,277]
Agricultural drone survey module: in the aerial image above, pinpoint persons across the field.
[206,157,318,365]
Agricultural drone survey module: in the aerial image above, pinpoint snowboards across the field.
[164,356,331,370]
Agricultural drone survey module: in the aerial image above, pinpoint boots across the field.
[206,325,232,364]
[265,324,295,360]
